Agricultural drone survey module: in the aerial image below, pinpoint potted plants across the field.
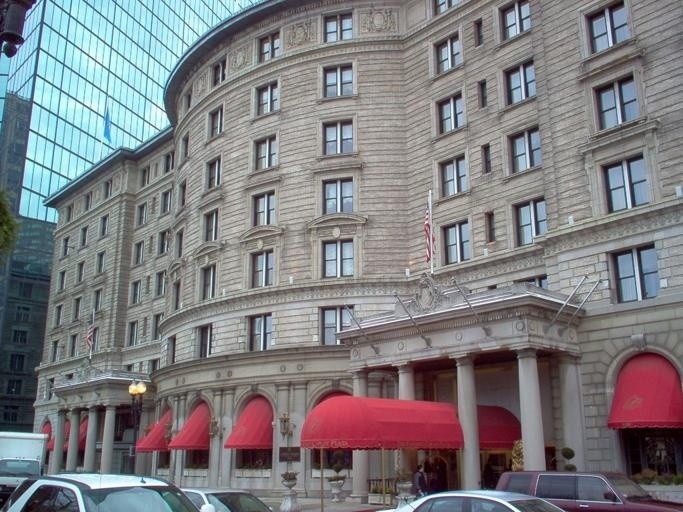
[367,484,395,506]
[327,475,347,501]
[281,471,300,490]
[630,468,681,503]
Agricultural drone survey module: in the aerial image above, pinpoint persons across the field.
[414,464,427,499]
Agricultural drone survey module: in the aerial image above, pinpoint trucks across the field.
[0,431,49,501]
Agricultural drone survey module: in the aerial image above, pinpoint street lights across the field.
[128,377,147,471]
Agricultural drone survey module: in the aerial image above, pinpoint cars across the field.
[1,472,274,512]
